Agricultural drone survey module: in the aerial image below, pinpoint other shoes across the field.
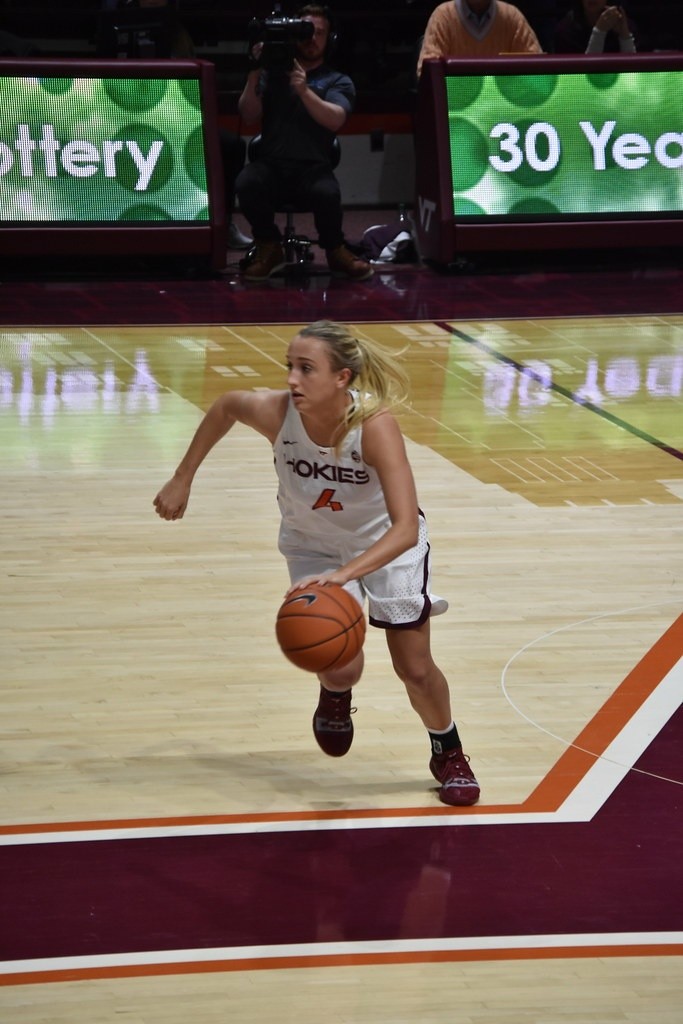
[245,225,288,280]
[324,246,375,280]
[224,223,253,249]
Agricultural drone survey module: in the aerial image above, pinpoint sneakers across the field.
[312,682,357,757]
[429,742,480,806]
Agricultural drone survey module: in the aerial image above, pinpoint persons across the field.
[153,319,480,805]
[417,0,543,79]
[235,7,372,280]
[554,0,637,55]
[111,0,253,249]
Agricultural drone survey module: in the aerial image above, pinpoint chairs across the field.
[239,129,340,263]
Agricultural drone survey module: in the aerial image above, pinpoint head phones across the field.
[327,28,336,42]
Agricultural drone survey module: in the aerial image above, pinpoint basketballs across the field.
[274,582,366,674]
